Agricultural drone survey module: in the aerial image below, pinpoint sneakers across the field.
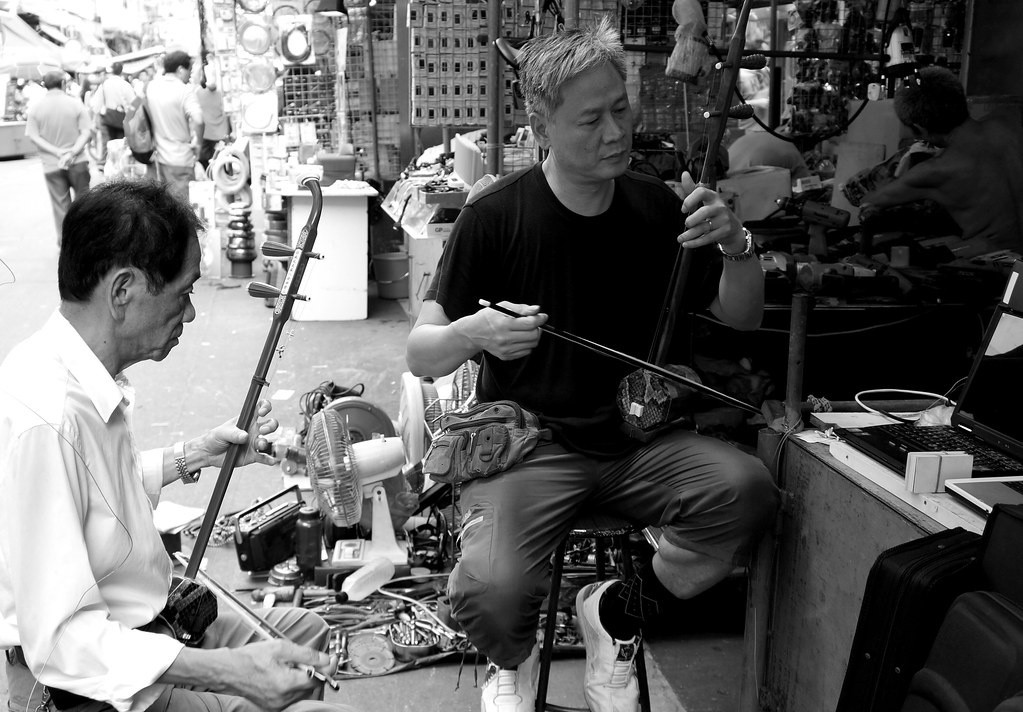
[576,578,639,711]
[480,637,540,712]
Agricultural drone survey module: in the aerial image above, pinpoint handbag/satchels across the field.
[422,401,539,483]
[103,105,126,129]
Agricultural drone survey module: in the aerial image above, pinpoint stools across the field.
[536,516,650,712]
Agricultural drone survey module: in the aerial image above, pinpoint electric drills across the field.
[771,198,852,256]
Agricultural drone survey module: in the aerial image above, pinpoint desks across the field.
[281,181,379,323]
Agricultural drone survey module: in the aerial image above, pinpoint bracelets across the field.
[174,441,201,484]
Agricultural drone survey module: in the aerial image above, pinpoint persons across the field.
[725,127,816,186]
[860,65,1023,243]
[405,18,776,712]
[24,50,230,249]
[0,180,340,712]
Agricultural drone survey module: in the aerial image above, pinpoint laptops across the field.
[832,301,1023,479]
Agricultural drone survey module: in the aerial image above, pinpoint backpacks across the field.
[124,97,154,164]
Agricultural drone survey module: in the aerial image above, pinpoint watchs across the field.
[719,227,756,261]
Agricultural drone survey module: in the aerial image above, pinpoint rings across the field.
[706,219,713,231]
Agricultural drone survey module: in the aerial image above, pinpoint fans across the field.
[305,408,408,567]
[398,360,481,530]
